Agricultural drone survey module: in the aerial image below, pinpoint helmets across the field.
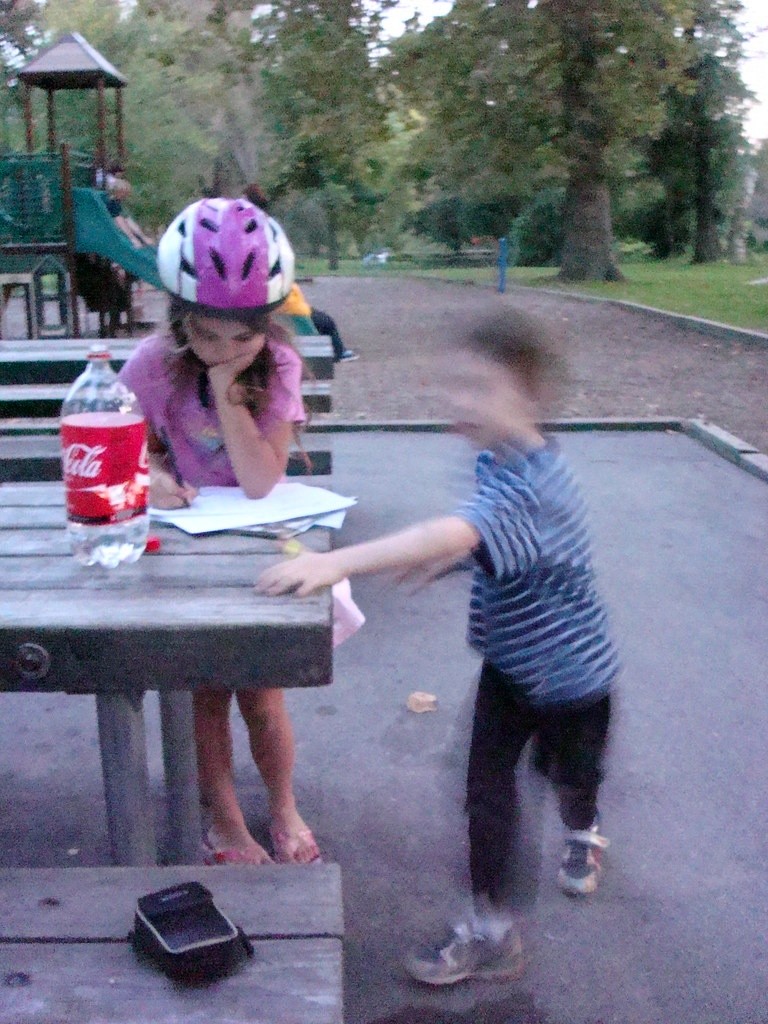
[155,196,297,318]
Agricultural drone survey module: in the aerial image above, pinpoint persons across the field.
[253,307,623,983]
[105,159,155,247]
[75,252,140,338]
[117,199,325,867]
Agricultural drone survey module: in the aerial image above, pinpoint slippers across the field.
[268,801,320,866]
[200,824,276,865]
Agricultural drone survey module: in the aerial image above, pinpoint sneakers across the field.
[335,350,360,364]
[404,919,524,986]
[555,810,610,898]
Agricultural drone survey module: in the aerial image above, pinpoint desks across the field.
[0,335,335,387]
[0,482,333,869]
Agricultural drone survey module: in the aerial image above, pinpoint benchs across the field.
[0,383,334,482]
[0,863,345,1023]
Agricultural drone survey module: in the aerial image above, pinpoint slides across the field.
[75,186,319,336]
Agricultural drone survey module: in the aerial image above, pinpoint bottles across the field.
[62,339,150,571]
